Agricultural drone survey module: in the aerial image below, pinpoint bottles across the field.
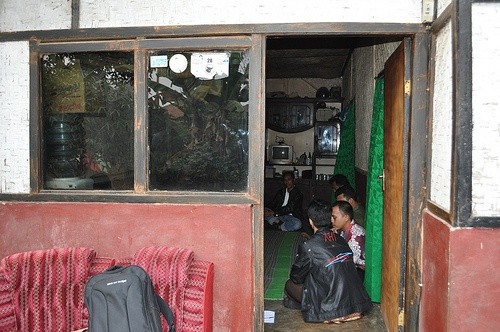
[44,114,80,176]
[307,152,312,166]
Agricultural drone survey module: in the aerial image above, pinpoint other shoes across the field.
[283,297,302,310]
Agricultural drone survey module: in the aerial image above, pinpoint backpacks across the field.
[84,265,176,332]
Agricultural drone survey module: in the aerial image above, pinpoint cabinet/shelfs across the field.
[265,98,345,211]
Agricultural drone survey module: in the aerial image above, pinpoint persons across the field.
[330,173,350,193]
[334,186,366,227]
[283,199,374,323]
[329,200,366,282]
[264,171,304,232]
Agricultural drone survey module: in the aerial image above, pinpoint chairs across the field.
[118,246,214,332]
[0,245,115,332]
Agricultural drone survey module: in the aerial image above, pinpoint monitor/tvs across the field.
[269,145,293,165]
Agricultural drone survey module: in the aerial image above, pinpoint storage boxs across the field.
[44,58,110,117]
